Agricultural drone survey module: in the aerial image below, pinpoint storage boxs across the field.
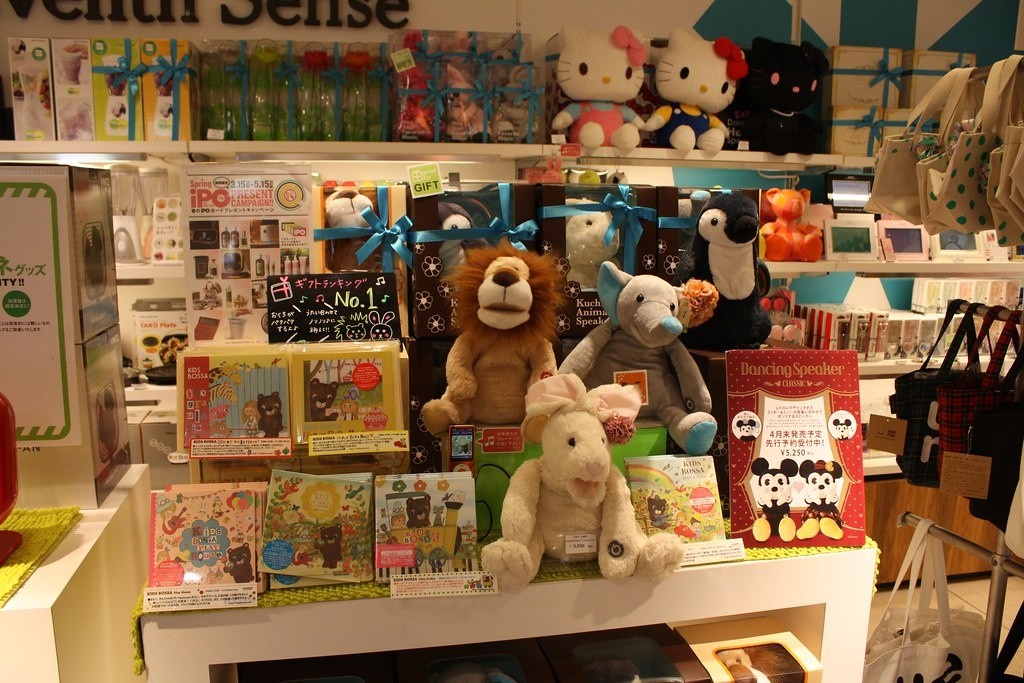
[0,161,120,343]
[408,180,760,340]
[0,323,132,510]
[92,37,144,142]
[141,397,190,489]
[312,185,409,338]
[794,278,1020,363]
[126,405,155,464]
[51,37,97,141]
[140,39,200,140]
[7,36,57,141]
[881,109,914,147]
[827,45,903,108]
[899,50,976,110]
[824,104,884,157]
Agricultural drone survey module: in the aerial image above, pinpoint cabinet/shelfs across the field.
[0,138,1024,588]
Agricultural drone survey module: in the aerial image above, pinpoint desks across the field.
[130,533,881,683]
[0,464,152,683]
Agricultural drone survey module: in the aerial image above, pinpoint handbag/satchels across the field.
[936,306,1022,501]
[862,54,1024,248]
[967,345,1024,559]
[987,601,1023,683]
[860,517,985,683]
[890,298,984,487]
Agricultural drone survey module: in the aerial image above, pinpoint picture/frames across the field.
[823,219,878,260]
[877,221,929,262]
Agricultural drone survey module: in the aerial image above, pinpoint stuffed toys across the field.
[419,239,564,446]
[482,375,684,591]
[552,27,648,150]
[744,37,830,155]
[716,643,805,683]
[324,188,385,275]
[668,193,771,353]
[565,197,620,292]
[437,194,493,277]
[558,257,721,456]
[645,28,749,152]
[675,190,712,270]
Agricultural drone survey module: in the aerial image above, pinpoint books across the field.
[724,349,867,550]
[621,454,727,554]
[144,339,478,605]
